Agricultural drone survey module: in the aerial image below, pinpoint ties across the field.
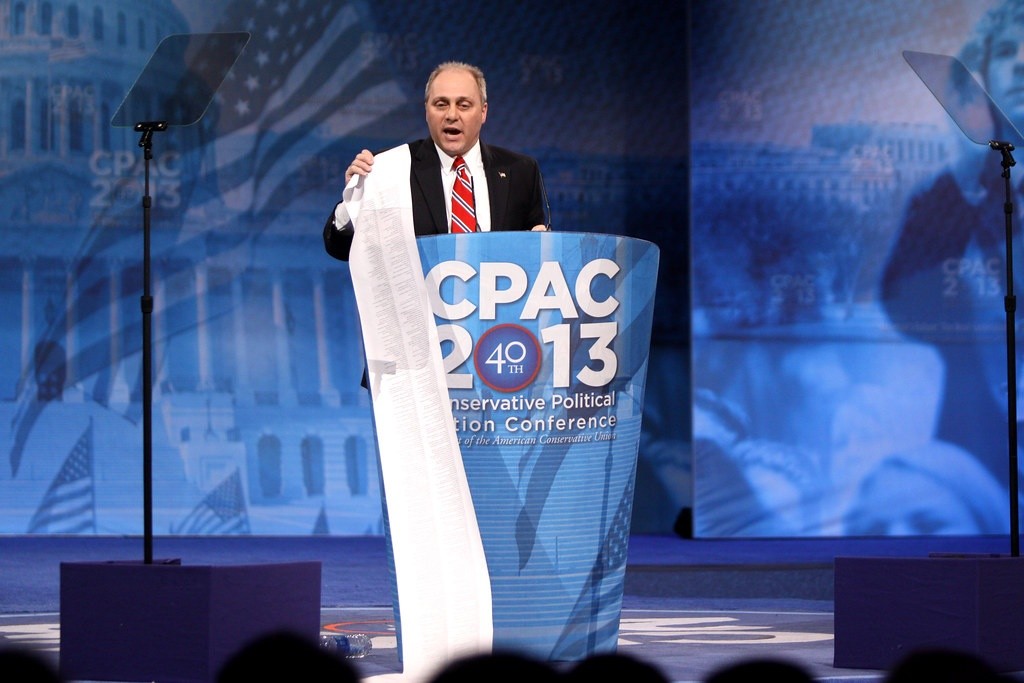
[451,157,477,234]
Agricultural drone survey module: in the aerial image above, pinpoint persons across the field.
[323,63,553,390]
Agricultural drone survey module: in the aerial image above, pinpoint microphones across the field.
[538,172,553,232]
[471,176,482,233]
